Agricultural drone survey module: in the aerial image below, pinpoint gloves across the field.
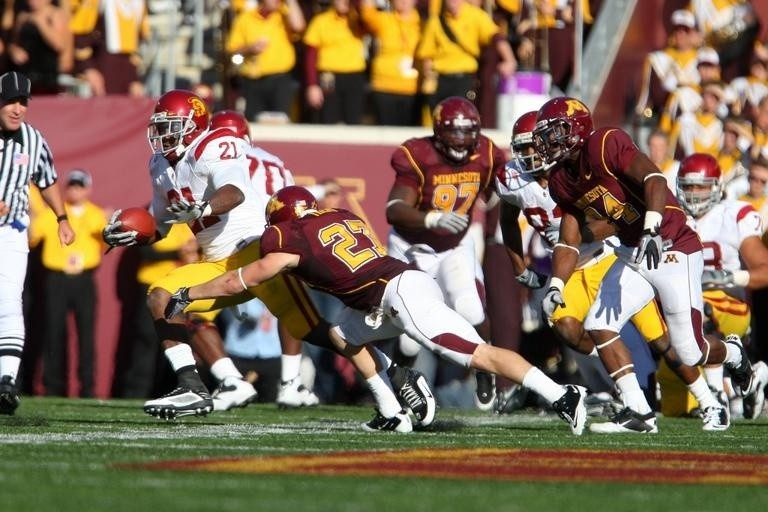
[540,227,560,245]
[634,211,663,270]
[164,200,212,224]
[516,268,548,289]
[541,277,566,328]
[424,209,469,235]
[103,209,138,255]
[164,287,191,318]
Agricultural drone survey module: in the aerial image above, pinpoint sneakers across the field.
[552,384,587,436]
[724,334,752,391]
[143,386,213,420]
[496,381,530,414]
[474,372,497,411]
[590,407,658,434]
[211,376,258,412]
[0,375,20,416]
[689,385,730,418]
[361,408,413,433]
[700,405,730,432]
[276,376,320,407]
[739,362,768,420]
[397,369,435,427]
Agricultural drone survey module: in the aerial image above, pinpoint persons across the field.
[1,0,158,103]
[492,110,733,433]
[217,295,282,403]
[25,169,109,398]
[651,150,767,418]
[118,223,202,398]
[1,70,76,414]
[184,108,321,414]
[220,0,595,124]
[160,185,589,439]
[101,87,438,428]
[380,95,515,411]
[632,0,767,207]
[531,96,755,436]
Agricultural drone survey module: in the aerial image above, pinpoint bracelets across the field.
[56,214,67,223]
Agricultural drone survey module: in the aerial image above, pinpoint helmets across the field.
[211,111,254,148]
[432,96,481,166]
[266,186,318,226]
[511,110,546,176]
[147,90,210,164]
[677,153,724,219]
[533,97,594,172]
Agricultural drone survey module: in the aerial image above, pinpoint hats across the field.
[0,72,32,101]
[69,170,90,186]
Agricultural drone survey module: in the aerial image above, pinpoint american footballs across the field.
[115,208,157,246]
